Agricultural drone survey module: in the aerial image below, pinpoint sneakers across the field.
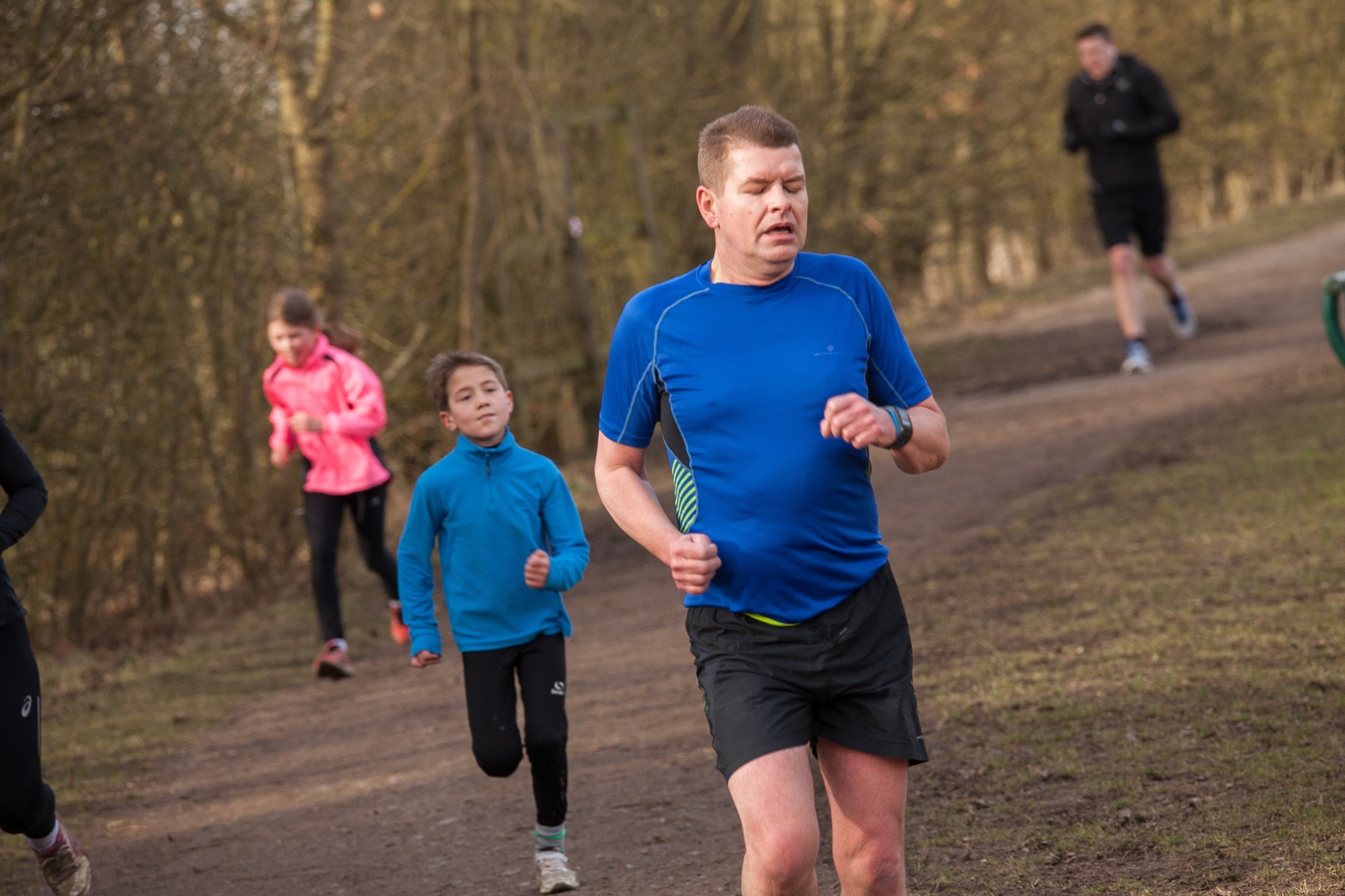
[389,605,409,644]
[314,638,355,679]
[534,847,580,893]
[24,814,92,896]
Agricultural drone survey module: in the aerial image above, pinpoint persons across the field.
[594,104,952,896]
[1058,21,1197,376]
[0,416,92,896]
[262,290,410,679]
[398,349,590,894]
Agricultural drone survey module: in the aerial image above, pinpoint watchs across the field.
[886,406,913,453]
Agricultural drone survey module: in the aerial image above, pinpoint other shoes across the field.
[1121,342,1150,374]
[1165,290,1196,340]
